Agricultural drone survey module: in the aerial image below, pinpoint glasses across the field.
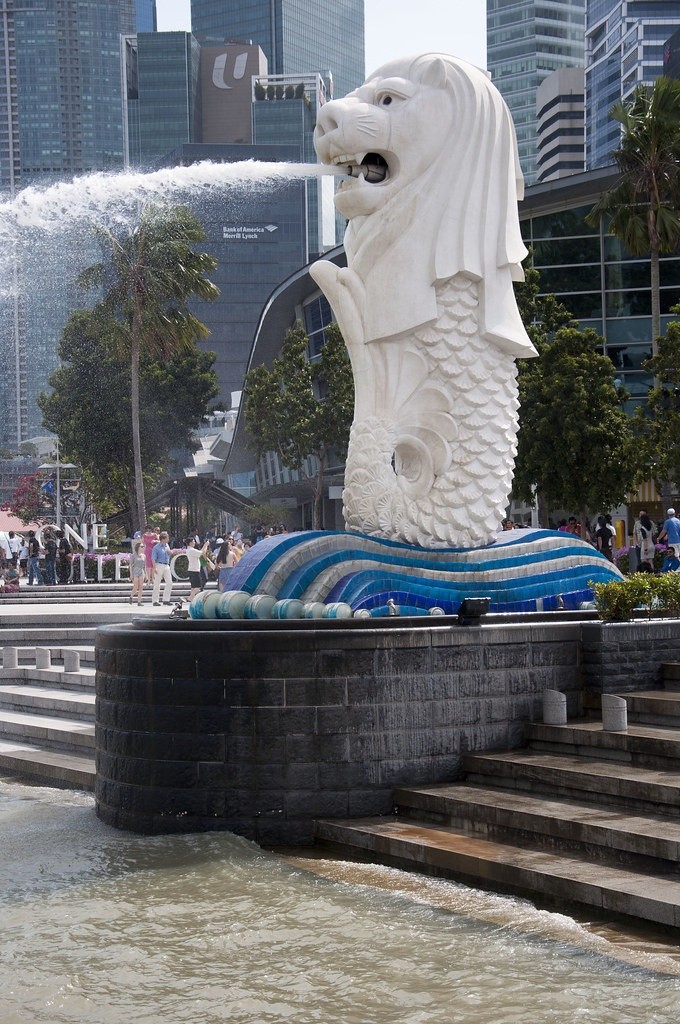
[571,523,575,525]
[229,538,233,540]
[139,546,144,548]
[666,551,671,553]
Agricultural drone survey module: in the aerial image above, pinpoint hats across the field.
[667,508,676,514]
[216,538,224,544]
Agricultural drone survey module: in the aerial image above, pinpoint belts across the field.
[159,562,167,564]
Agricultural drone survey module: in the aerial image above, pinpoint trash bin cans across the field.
[628,545,640,574]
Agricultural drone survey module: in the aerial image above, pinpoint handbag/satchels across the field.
[11,552,18,560]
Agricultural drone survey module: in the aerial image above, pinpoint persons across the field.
[56,531,72,584]
[42,534,58,585]
[0,560,20,593]
[122,523,251,597]
[26,531,44,585]
[0,531,21,569]
[129,543,149,606]
[185,537,210,603]
[503,508,680,575]
[151,531,174,606]
[249,524,326,545]
[17,542,29,578]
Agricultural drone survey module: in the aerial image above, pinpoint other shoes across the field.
[153,602,160,606]
[130,596,132,604]
[163,601,173,605]
[138,602,144,606]
